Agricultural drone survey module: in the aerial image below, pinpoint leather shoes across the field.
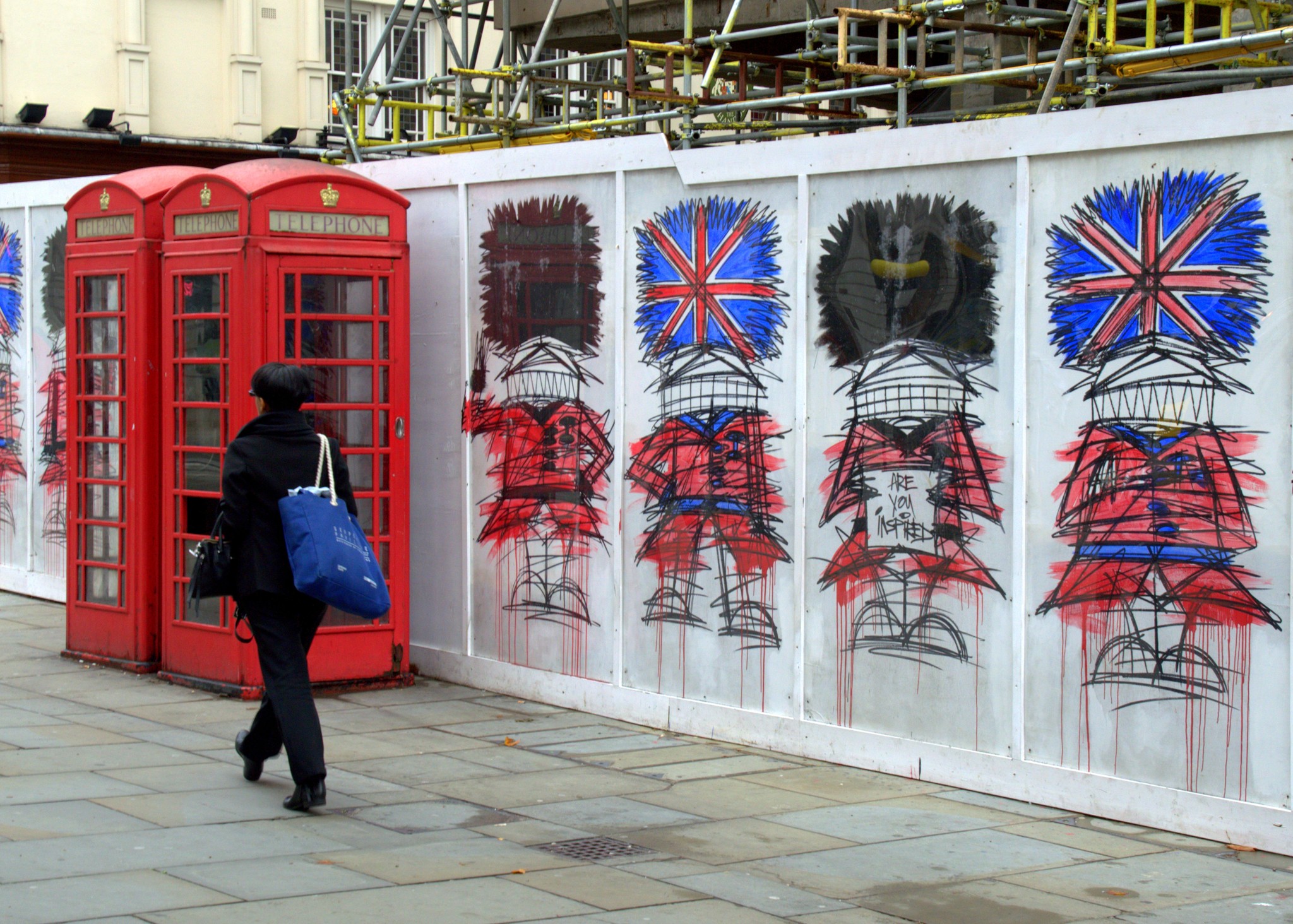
[235,730,264,781]
[283,777,327,811]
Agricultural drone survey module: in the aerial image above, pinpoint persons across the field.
[218,360,357,810]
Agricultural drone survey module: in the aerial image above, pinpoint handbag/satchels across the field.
[278,433,391,620]
[186,511,243,618]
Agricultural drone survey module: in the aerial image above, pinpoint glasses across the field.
[249,389,257,397]
